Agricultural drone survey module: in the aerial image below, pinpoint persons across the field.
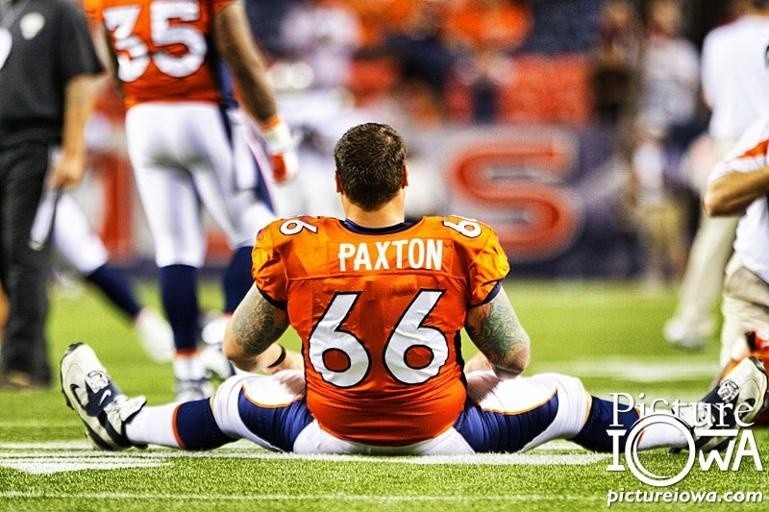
[60,123,769,455]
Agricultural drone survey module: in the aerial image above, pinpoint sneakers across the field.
[174,373,211,404]
[60,341,148,452]
[668,355,768,454]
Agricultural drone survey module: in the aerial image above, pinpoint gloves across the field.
[259,117,301,185]
